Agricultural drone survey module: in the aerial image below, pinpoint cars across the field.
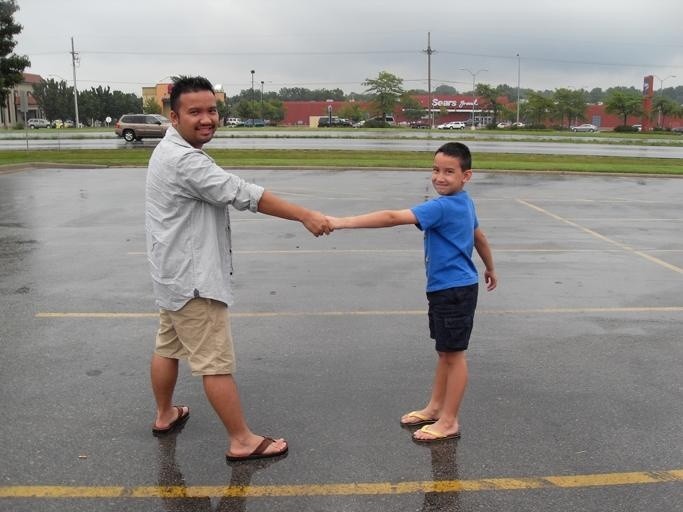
[115,114,171,142]
[571,124,598,132]
[244,118,264,127]
[632,125,642,132]
[497,122,525,128]
[27,119,70,129]
[411,121,464,130]
[227,118,240,125]
[318,116,396,128]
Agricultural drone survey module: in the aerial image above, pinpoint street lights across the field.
[261,80,264,105]
[516,53,520,127]
[250,69,255,117]
[460,68,488,129]
[672,127,683,132]
[649,75,677,128]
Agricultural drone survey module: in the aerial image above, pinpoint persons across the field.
[146,76,324,462]
[319,140,497,442]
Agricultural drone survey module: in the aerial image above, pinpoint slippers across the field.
[400,409,436,426]
[152,405,189,433]
[225,435,288,461]
[411,424,461,443]
